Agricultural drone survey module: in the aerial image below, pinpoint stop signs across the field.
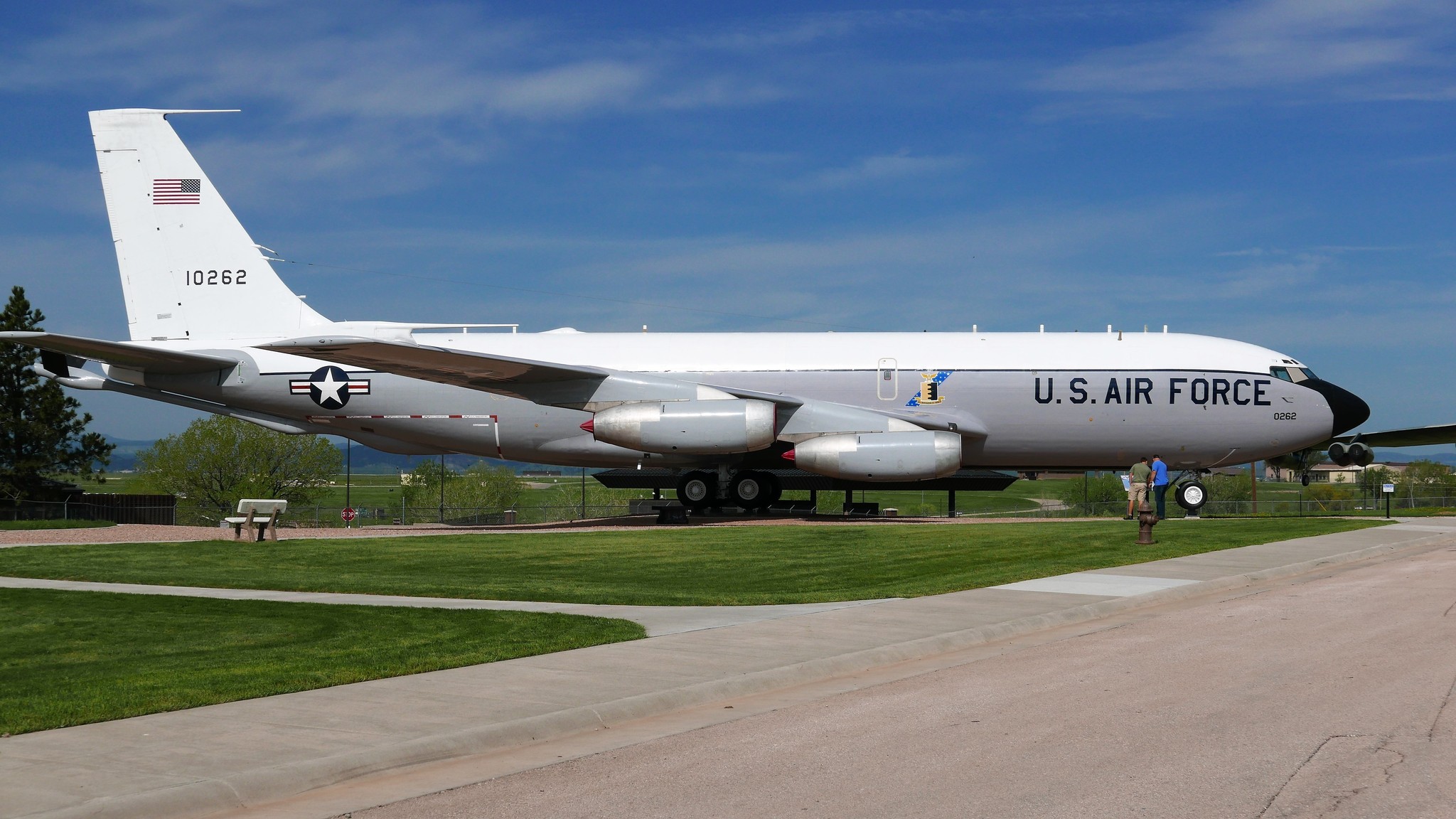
[341,508,355,521]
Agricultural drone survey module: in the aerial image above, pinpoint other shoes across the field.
[1158,516,1163,520]
[1137,516,1140,520]
[1124,515,1133,519]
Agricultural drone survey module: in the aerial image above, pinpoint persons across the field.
[1148,454,1169,519]
[1123,457,1152,520]
[1268,474,1273,479]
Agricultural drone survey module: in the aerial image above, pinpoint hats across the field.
[1152,455,1160,459]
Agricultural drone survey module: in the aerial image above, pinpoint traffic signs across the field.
[1354,507,1363,509]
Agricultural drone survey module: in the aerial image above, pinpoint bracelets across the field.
[1149,482,1152,484]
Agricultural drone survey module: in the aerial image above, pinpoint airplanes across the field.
[0,108,1372,512]
[1302,423,1456,467]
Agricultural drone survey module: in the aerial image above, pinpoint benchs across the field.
[225,499,287,543]
